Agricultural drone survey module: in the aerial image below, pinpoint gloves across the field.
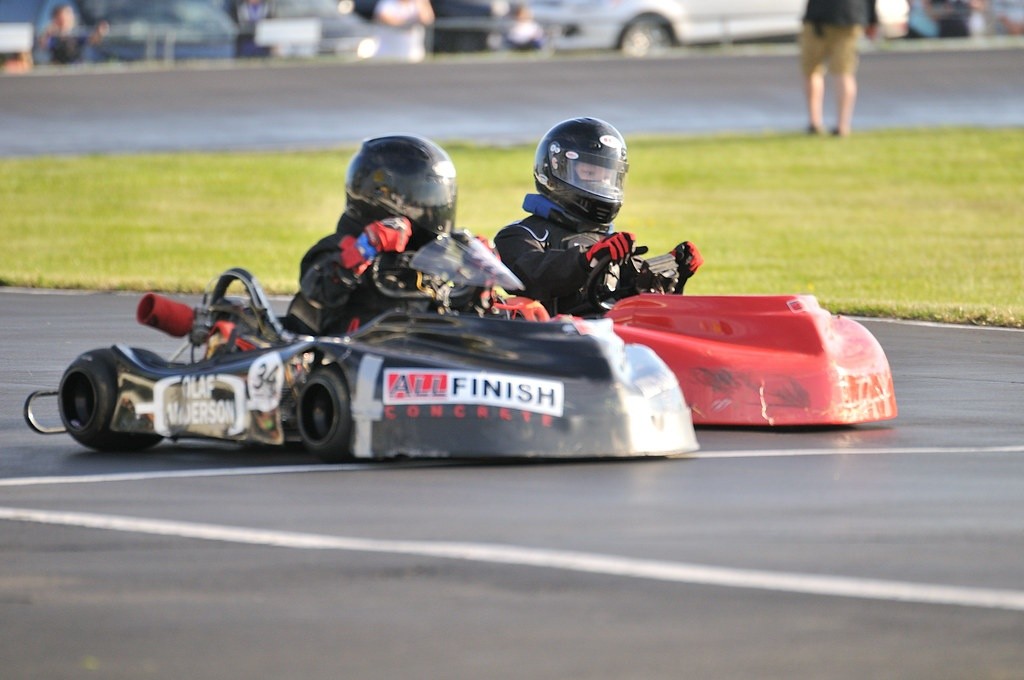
[487,295,551,323]
[667,241,704,295]
[582,232,636,272]
[340,217,413,279]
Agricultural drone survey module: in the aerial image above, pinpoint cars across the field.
[1,0,916,64]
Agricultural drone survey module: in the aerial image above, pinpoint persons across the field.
[907,0,974,39]
[40,6,110,63]
[796,0,879,136]
[503,6,545,52]
[493,117,704,320]
[299,135,551,337]
[373,0,434,60]
[227,0,277,58]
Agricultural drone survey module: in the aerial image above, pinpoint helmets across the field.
[344,135,460,252]
[532,117,629,223]
